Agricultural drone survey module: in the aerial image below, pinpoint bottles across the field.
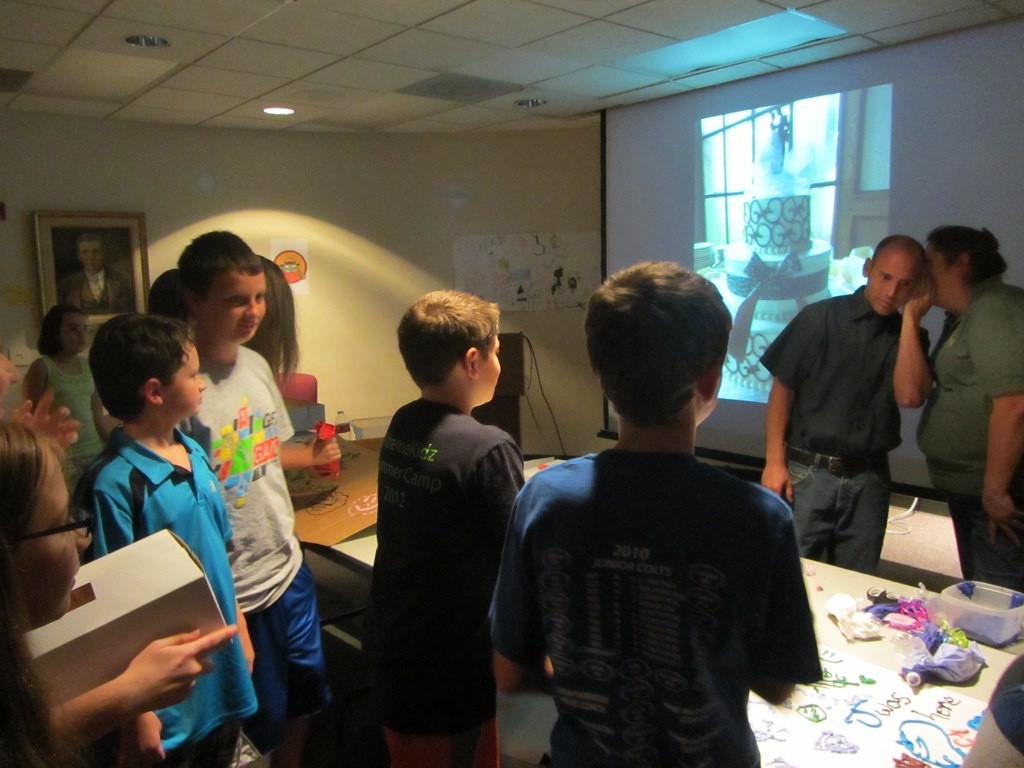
[335,411,353,462]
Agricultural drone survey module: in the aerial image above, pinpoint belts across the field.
[785,446,890,478]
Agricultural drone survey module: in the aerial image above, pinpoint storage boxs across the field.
[23,527,228,710]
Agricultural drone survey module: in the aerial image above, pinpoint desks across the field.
[292,437,1023,768]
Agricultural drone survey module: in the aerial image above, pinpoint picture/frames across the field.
[31,208,150,333]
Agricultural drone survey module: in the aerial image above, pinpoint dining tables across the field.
[696,258,868,403]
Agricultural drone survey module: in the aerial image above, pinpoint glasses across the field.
[7,508,94,549]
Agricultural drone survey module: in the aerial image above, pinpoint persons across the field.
[146,256,346,472]
[68,312,254,768]
[174,232,350,768]
[63,234,135,312]
[758,235,935,577]
[0,353,238,768]
[916,225,1024,592]
[488,259,825,766]
[20,303,115,522]
[363,292,528,766]
